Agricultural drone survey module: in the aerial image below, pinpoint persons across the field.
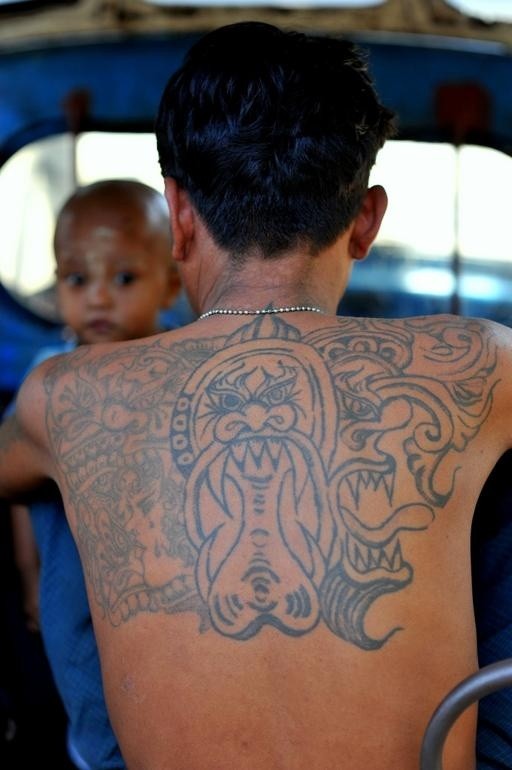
[10,178,183,627]
[0,22,512,769]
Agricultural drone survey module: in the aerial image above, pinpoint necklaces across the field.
[195,307,323,317]
